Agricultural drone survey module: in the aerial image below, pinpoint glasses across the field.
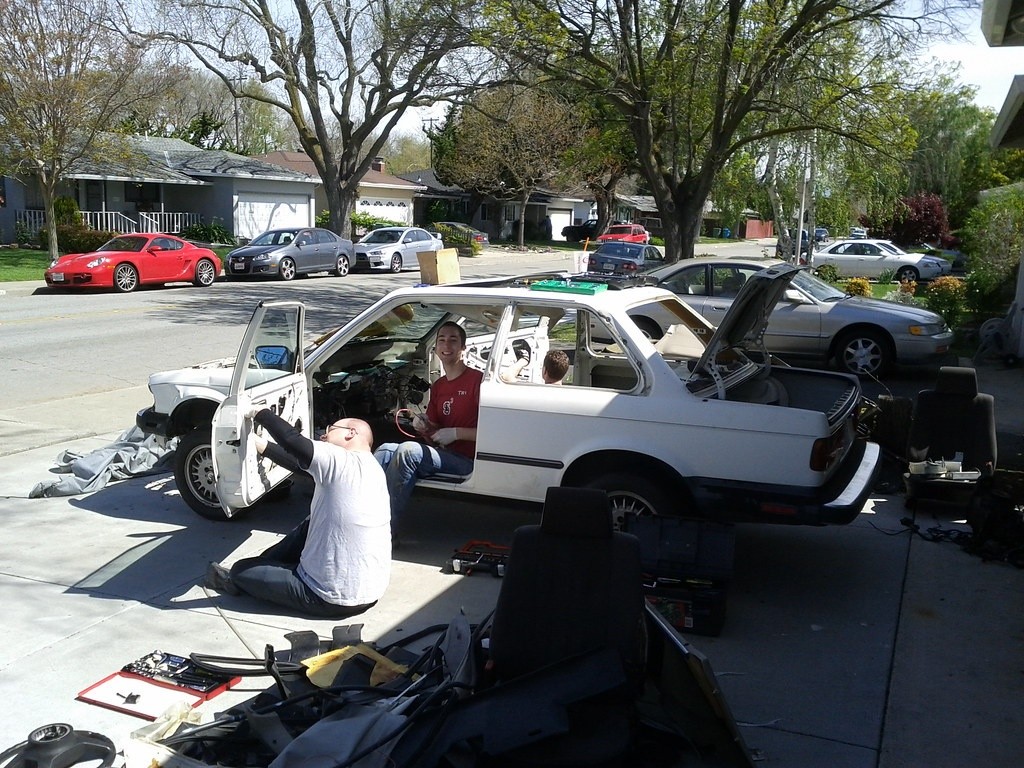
[325,425,359,434]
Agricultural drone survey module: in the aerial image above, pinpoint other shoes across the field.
[206,562,240,596]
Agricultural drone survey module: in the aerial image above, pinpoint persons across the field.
[206,405,392,618]
[504,346,570,384]
[374,322,484,549]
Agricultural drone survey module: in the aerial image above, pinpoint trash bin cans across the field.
[723,227,730,238]
[713,228,721,237]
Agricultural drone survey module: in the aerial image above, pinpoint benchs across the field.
[662,279,689,295]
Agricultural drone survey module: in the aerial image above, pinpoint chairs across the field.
[282,237,291,245]
[160,240,170,250]
[902,367,997,499]
[488,487,643,768]
[721,273,746,298]
[302,235,310,244]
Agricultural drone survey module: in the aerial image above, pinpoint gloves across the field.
[412,414,430,432]
[430,427,457,448]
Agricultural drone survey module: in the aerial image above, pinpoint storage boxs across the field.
[78,648,241,721]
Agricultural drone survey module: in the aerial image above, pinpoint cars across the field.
[586,238,663,274]
[44,231,223,294]
[806,238,952,284]
[775,226,811,255]
[643,254,956,377]
[224,225,357,280]
[849,226,867,239]
[815,228,829,242]
[353,226,444,274]
[424,221,490,257]
[132,260,884,534]
[596,223,649,245]
